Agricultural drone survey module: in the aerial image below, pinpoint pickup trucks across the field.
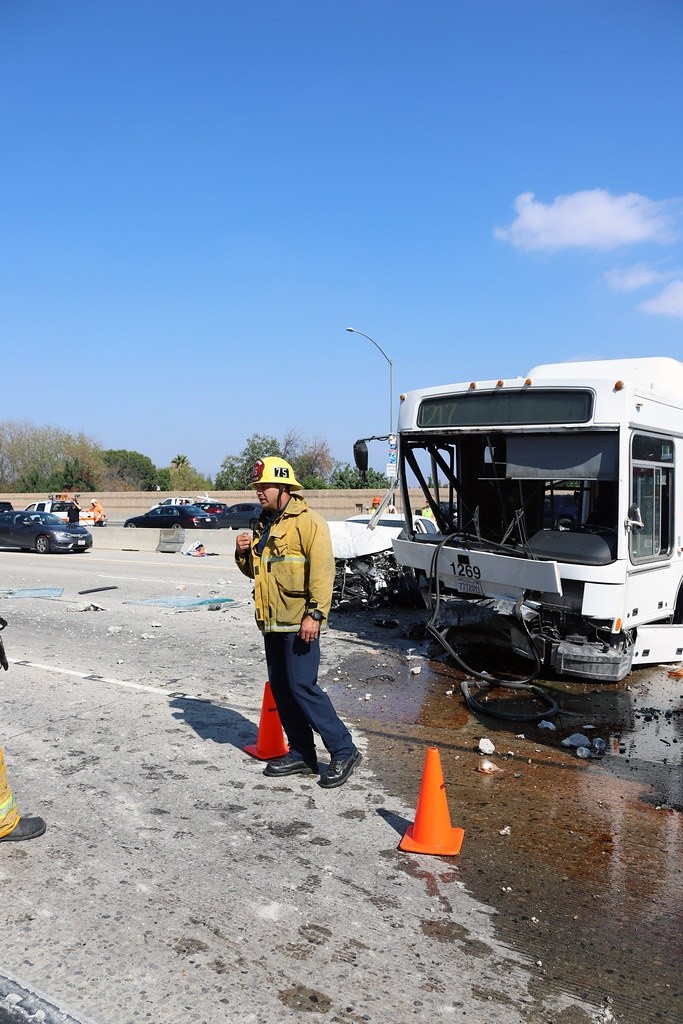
[23,493,95,527]
[150,497,193,510]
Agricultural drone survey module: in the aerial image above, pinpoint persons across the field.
[236,456,363,788]
[86,498,104,527]
[368,497,437,523]
[0,618,46,841]
[65,499,82,527]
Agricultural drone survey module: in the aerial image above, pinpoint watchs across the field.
[309,612,321,621]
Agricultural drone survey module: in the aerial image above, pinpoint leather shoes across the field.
[264,754,318,777]
[320,748,363,788]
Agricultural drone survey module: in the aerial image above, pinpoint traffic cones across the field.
[398,746,466,856]
[243,679,290,760]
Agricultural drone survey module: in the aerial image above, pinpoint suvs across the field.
[0,501,14,524]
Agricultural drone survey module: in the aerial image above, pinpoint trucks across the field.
[354,358,683,684]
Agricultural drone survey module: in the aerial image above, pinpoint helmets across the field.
[90,498,97,503]
[248,456,305,489]
[373,497,382,504]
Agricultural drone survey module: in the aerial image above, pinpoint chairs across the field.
[16,517,25,523]
[34,517,41,523]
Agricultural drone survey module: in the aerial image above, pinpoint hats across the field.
[71,500,79,505]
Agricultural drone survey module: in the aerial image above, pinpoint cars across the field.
[346,514,439,534]
[217,502,265,531]
[0,510,92,554]
[192,502,229,516]
[124,504,220,529]
